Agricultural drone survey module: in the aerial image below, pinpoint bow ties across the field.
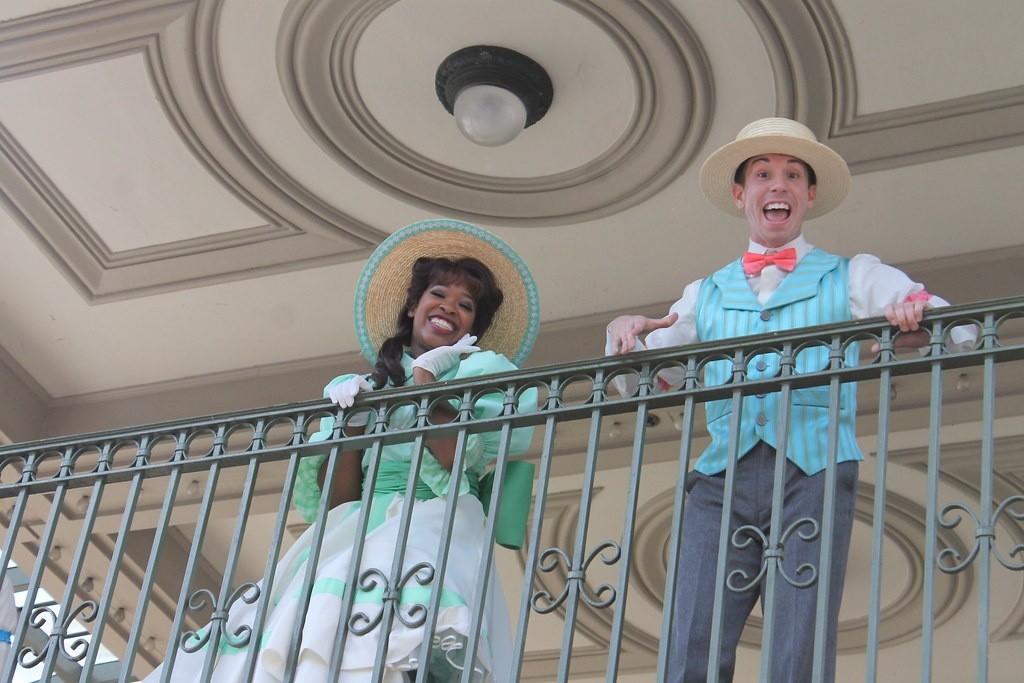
[743,247,796,273]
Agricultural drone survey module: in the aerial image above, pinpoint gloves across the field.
[329,374,373,428]
[411,332,480,376]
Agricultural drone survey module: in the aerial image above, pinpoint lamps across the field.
[435,46,555,150]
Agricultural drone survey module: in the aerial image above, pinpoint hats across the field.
[352,219,543,370]
[699,117,852,222]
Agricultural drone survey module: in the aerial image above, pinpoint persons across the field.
[602,118,978,683]
[144,256,538,683]
